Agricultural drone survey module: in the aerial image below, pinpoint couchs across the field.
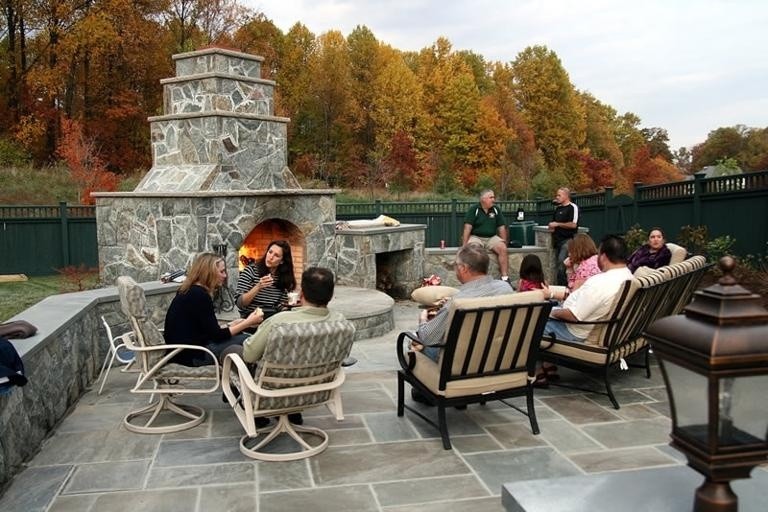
[540,254,717,411]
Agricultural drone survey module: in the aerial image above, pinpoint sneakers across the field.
[223,387,240,402]
[412,390,433,407]
[254,416,270,427]
[500,277,516,290]
[288,413,302,424]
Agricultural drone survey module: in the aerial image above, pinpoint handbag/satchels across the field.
[0,337,28,391]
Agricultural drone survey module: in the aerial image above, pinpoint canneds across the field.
[440,240,445,249]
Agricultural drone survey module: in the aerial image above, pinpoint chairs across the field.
[666,243,687,265]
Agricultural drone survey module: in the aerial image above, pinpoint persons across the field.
[626,227,671,274]
[218,267,347,427]
[548,187,580,287]
[234,239,358,366]
[532,234,635,389]
[163,253,263,403]
[411,248,514,410]
[462,189,516,292]
[518,254,548,292]
[531,234,603,307]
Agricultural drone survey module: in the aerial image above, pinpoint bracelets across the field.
[550,288,554,299]
[558,223,560,227]
[225,322,232,327]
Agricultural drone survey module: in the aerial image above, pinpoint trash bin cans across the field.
[509,221,539,245]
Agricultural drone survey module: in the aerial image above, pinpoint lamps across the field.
[643,256,768,512]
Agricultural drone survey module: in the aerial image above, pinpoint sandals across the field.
[540,362,560,380]
[533,368,548,388]
